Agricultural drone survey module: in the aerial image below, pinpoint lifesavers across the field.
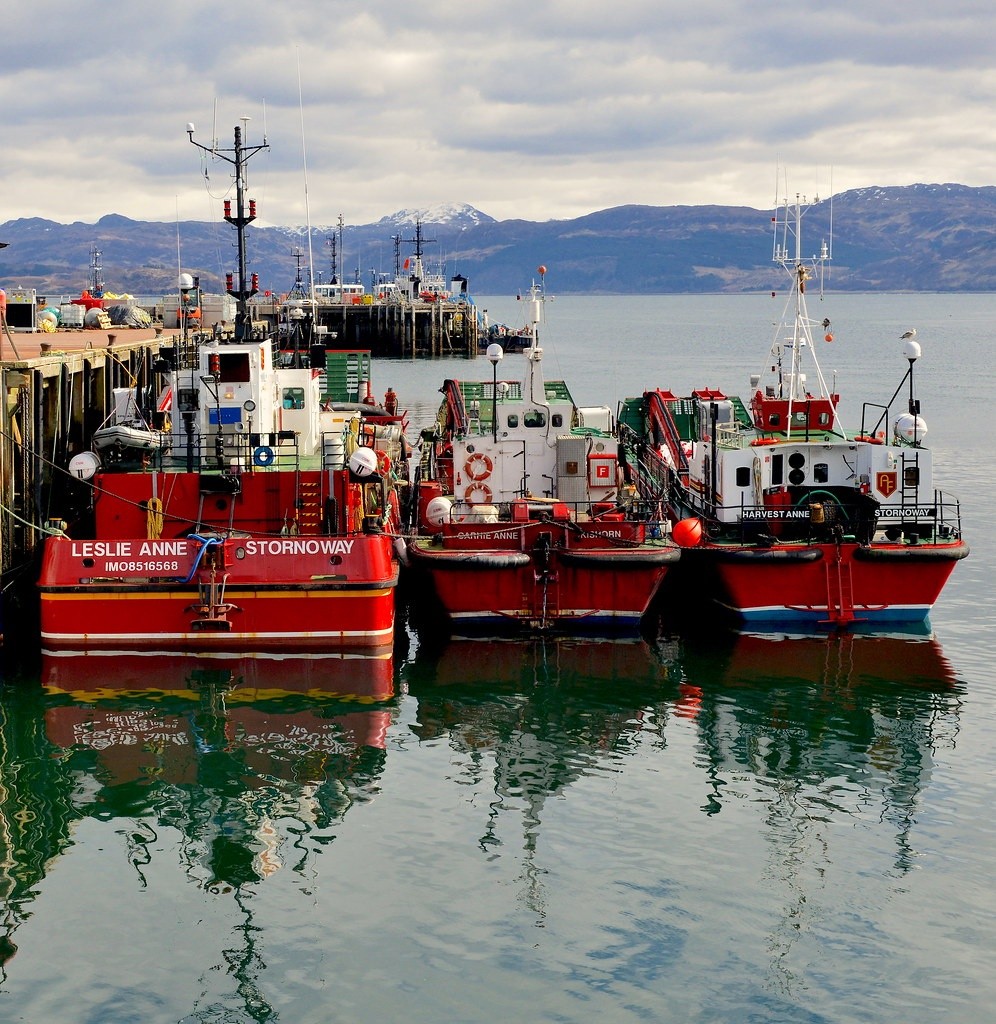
[465,452,493,481]
[363,427,374,448]
[253,446,274,467]
[464,482,493,508]
[854,435,883,446]
[751,437,780,446]
[372,450,391,476]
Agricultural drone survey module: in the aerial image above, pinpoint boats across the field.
[475,307,539,356]
[407,265,679,642]
[40,613,966,793]
[615,155,970,634]
[37,68,410,648]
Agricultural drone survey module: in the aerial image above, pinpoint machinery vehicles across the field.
[177,275,204,329]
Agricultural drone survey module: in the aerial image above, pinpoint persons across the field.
[215,319,230,341]
